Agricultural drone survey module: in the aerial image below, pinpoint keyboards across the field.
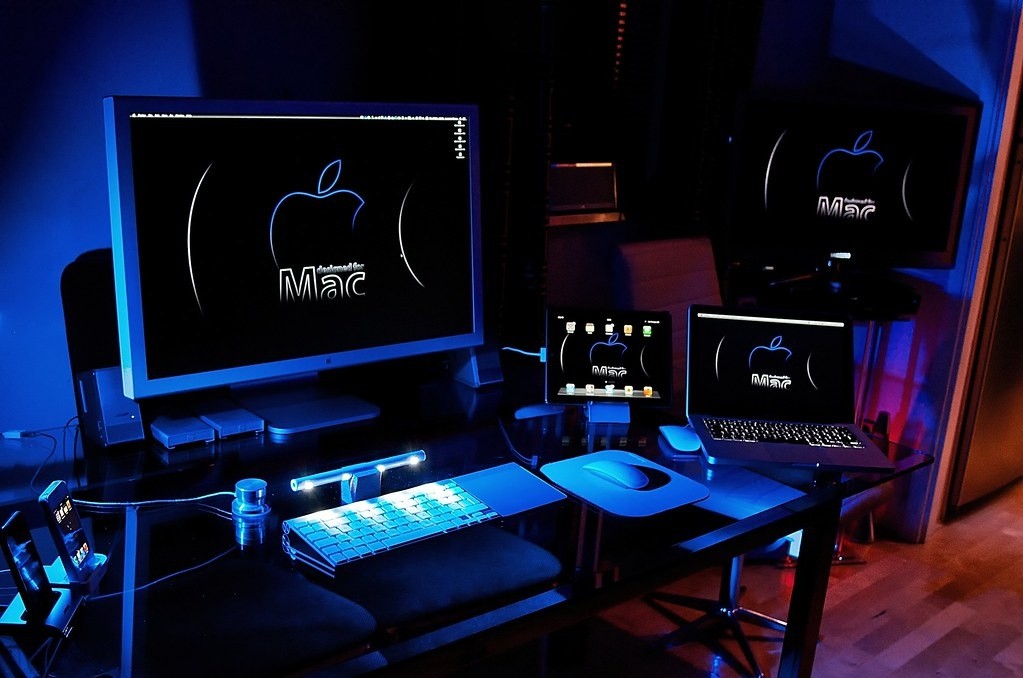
[281,479,500,569]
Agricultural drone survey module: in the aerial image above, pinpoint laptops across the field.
[685,304,891,474]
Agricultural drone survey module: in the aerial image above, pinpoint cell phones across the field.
[1,510,56,617]
[37,478,97,582]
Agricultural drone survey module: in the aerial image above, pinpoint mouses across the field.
[659,424,700,454]
[582,460,649,490]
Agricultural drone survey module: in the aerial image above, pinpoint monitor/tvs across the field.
[103,94,485,399]
[715,56,983,272]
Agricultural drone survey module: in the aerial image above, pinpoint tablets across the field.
[544,304,674,408]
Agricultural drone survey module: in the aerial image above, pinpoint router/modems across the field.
[150,408,266,450]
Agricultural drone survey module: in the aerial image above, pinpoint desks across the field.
[0,382,938,677]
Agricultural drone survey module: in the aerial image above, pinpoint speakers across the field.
[76,366,144,447]
[451,346,506,389]
